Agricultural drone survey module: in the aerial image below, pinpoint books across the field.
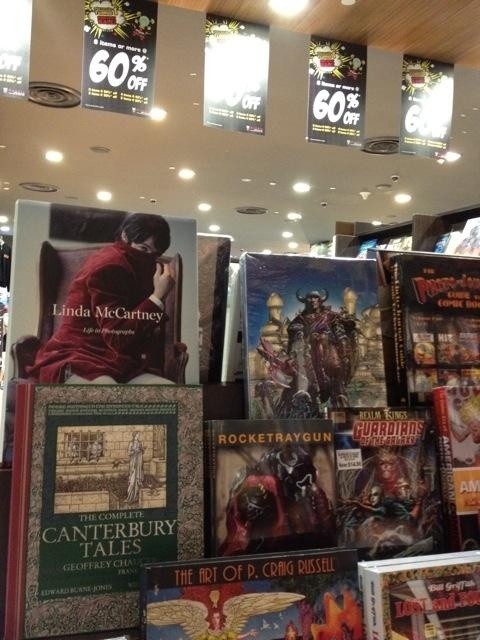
[1,211,478,640]
